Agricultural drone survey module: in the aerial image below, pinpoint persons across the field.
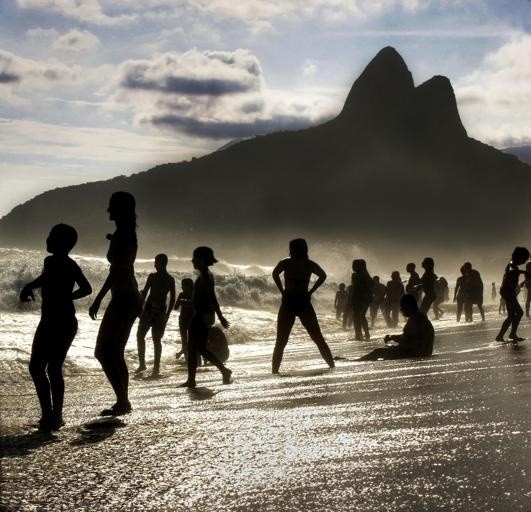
[406,263,422,308]
[174,278,194,367]
[495,246,529,343]
[453,266,469,322]
[334,283,346,322]
[492,282,497,304]
[433,277,448,320]
[272,238,335,374]
[499,296,506,314]
[89,191,143,416]
[351,259,375,342]
[369,276,391,329]
[175,246,233,388]
[343,285,353,331]
[463,262,485,322]
[20,224,92,435]
[135,254,175,374]
[413,257,438,314]
[384,271,404,328]
[525,255,531,317]
[332,295,434,362]
